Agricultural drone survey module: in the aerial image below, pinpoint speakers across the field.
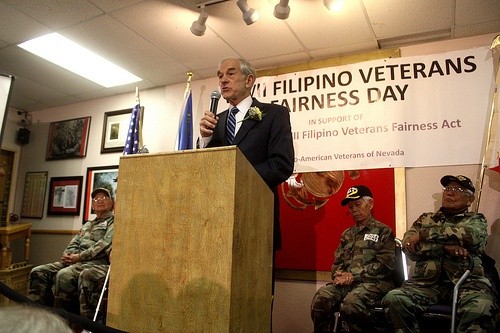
[18,128,30,144]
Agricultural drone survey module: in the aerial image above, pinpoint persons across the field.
[311,185,394,333]
[381,174,500,333]
[197,58,295,333]
[27,187,115,333]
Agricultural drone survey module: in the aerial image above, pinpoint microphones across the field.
[209,90,221,118]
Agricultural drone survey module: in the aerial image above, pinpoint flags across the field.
[122,103,141,155]
[484,35,500,176]
[177,88,194,150]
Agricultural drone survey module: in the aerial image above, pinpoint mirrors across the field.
[45,116,91,160]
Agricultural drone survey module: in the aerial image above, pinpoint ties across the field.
[226,107,240,146]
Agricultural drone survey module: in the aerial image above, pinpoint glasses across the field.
[94,196,110,200]
[442,185,472,195]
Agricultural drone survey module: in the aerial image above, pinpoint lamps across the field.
[190,0,344,36]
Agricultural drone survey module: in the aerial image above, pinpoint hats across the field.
[341,185,373,206]
[441,175,476,192]
[91,186,113,199]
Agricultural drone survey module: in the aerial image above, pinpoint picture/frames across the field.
[20,171,48,219]
[81,165,119,225]
[101,106,144,154]
[47,176,83,216]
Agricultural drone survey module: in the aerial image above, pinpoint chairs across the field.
[422,250,496,326]
[333,238,403,333]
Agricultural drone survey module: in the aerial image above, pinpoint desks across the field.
[0,223,32,270]
